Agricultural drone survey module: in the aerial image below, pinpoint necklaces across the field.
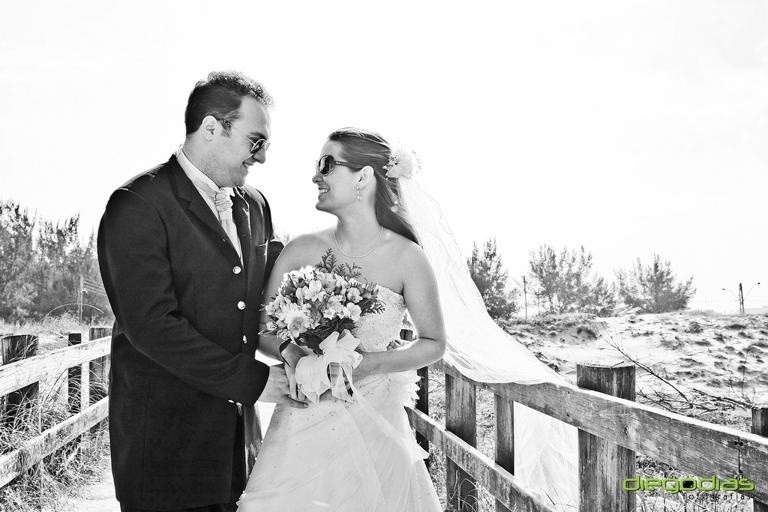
[333,226,384,258]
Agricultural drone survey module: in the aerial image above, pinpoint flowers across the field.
[254,246,386,370]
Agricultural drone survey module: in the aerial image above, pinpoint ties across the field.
[216,190,242,264]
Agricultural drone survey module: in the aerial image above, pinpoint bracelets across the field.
[279,340,286,360]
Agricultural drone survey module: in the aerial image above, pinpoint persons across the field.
[237,126,582,510]
[98,72,310,510]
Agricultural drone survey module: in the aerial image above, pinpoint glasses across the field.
[317,154,363,175]
[215,117,270,153]
